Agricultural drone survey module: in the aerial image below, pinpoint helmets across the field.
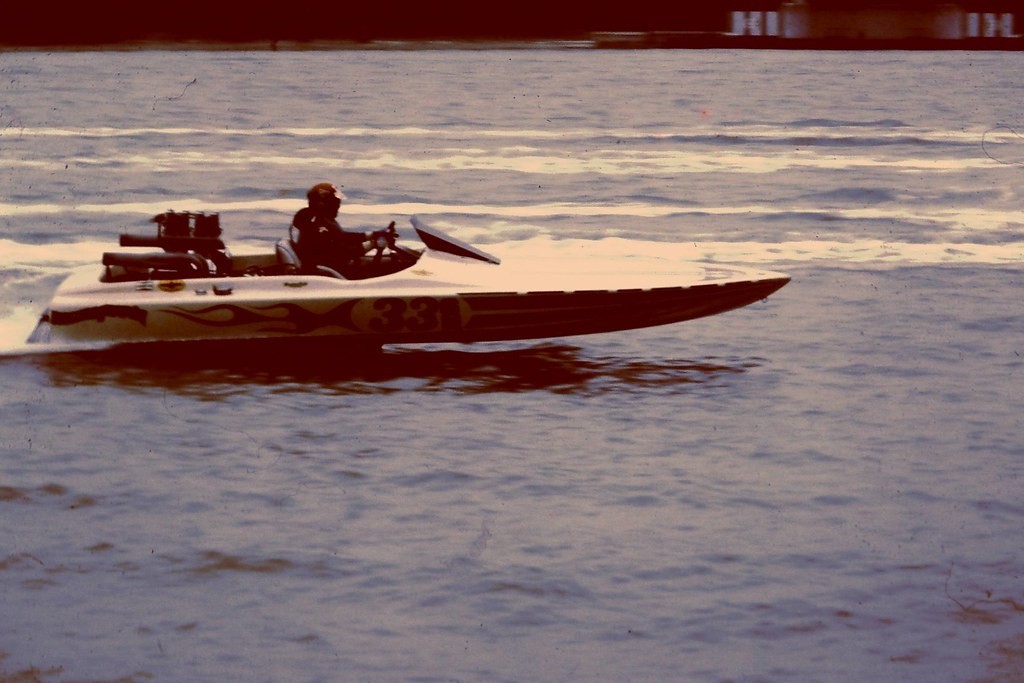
[307,183,347,207]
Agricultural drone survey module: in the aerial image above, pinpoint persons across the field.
[290,183,394,280]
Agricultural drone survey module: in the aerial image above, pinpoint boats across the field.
[46,209,792,343]
